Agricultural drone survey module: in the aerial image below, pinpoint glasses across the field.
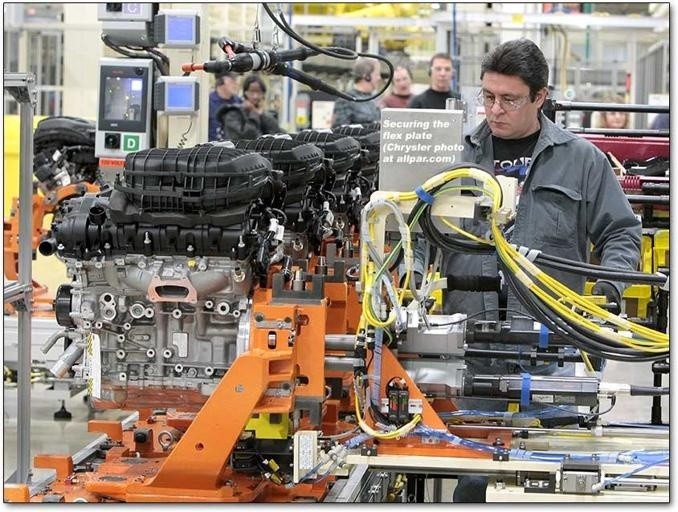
[476,87,530,110]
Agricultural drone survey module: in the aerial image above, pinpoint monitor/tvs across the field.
[94,58,154,159]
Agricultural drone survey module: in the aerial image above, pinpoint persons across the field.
[331,58,381,129]
[406,53,462,110]
[398,38,642,503]
[216,77,280,144]
[208,72,243,142]
[376,63,417,110]
[599,95,629,128]
